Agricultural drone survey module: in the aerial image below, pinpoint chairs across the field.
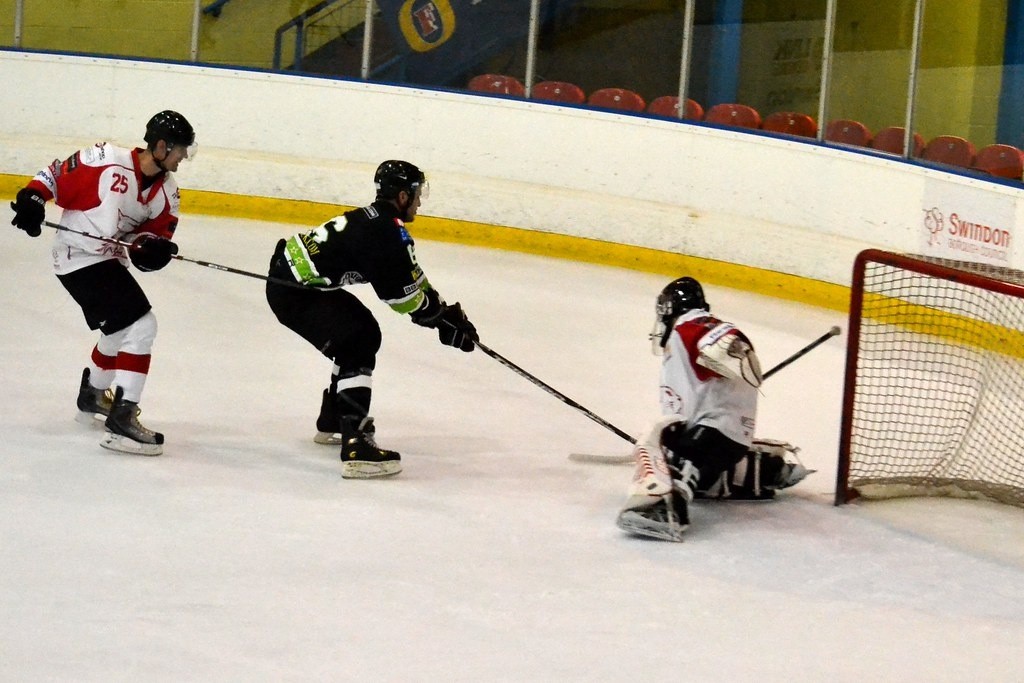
[586,87,646,112]
[467,73,524,97]
[972,143,1024,179]
[761,111,816,137]
[923,134,977,168]
[530,82,586,104]
[825,120,871,146]
[871,126,925,156]
[646,96,704,122]
[704,103,762,130]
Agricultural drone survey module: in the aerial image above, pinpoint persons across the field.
[612,275,817,545]
[8,109,196,454]
[263,158,482,480]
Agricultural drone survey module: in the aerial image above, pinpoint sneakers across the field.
[74,368,116,432]
[341,415,402,478]
[100,386,164,455]
[313,388,343,444]
[618,480,691,543]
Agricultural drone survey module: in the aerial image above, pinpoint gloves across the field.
[131,237,178,273]
[436,302,479,352]
[406,288,442,329]
[10,188,45,238]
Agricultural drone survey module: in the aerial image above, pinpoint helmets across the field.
[659,277,705,325]
[375,159,425,198]
[144,110,195,146]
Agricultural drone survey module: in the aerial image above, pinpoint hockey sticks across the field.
[472,340,675,446]
[566,324,842,468]
[7,199,321,295]
